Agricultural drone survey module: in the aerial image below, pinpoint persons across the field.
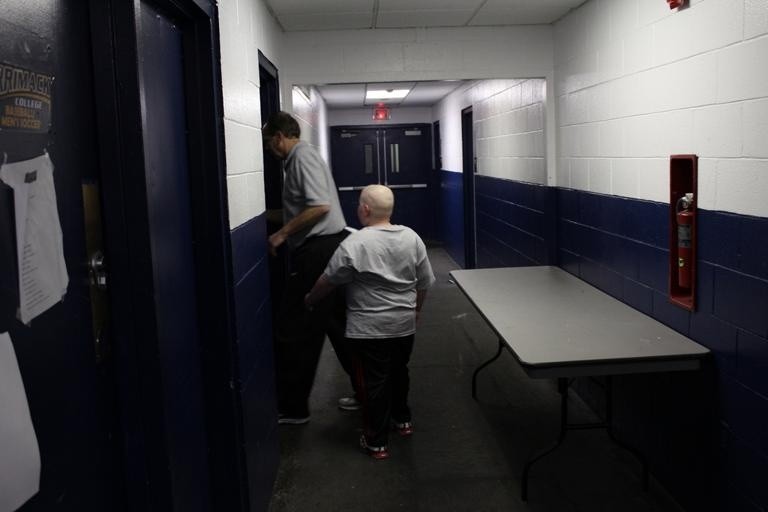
[262,111,360,425]
[303,184,436,460]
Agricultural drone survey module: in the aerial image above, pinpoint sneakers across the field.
[269,407,311,425]
[393,419,412,435]
[358,435,390,459]
[336,396,358,410]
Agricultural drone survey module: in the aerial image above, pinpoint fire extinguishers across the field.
[676,193,695,287]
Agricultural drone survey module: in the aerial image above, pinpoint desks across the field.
[448,263,710,505]
